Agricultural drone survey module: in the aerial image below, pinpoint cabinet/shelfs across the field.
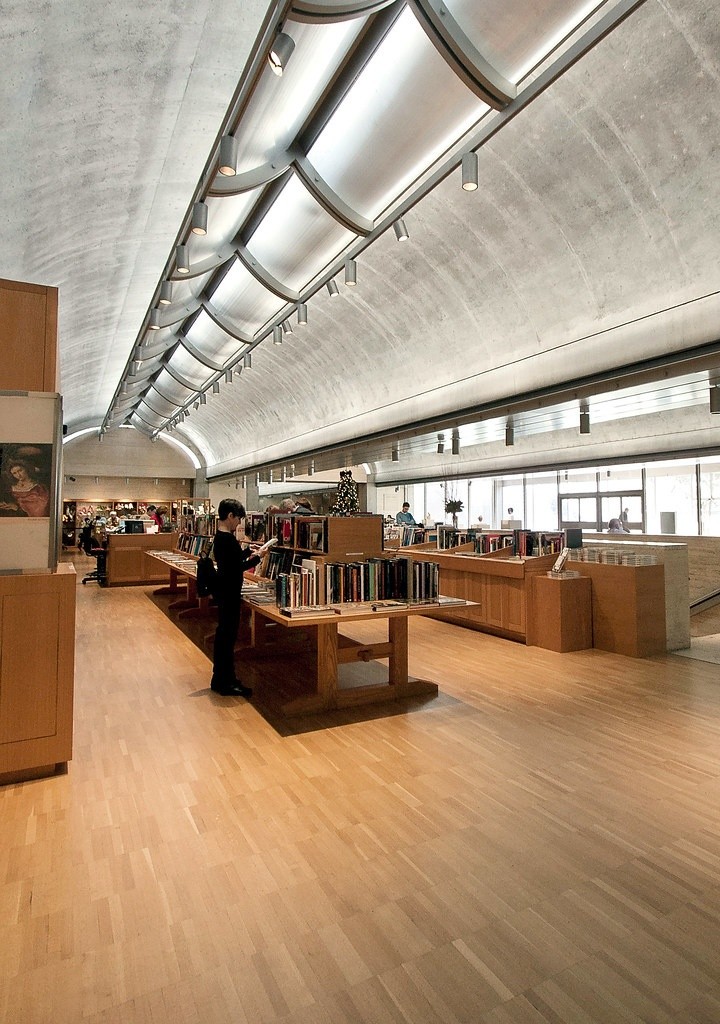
[0,498,665,775]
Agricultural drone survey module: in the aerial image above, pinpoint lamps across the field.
[345,260,356,286]
[160,281,172,305]
[326,280,339,297]
[191,202,207,235]
[268,33,295,76]
[282,320,292,334]
[393,215,409,241]
[149,308,160,330]
[218,136,236,177]
[243,460,314,489]
[710,379,720,414]
[392,405,590,462]
[176,244,190,274]
[167,354,251,432]
[274,326,282,344]
[462,153,479,191]
[99,346,142,442]
[298,304,307,325]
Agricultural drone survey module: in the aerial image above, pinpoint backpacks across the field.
[196,540,217,598]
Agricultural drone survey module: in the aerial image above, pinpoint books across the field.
[467,528,565,557]
[154,511,466,618]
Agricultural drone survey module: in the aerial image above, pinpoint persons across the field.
[266,498,313,512]
[150,506,167,530]
[619,508,629,521]
[140,505,156,520]
[607,519,623,533]
[397,502,416,524]
[108,511,119,526]
[210,498,268,696]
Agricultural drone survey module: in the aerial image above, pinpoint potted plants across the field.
[445,499,464,529]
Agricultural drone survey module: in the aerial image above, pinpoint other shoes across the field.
[210,678,252,696]
